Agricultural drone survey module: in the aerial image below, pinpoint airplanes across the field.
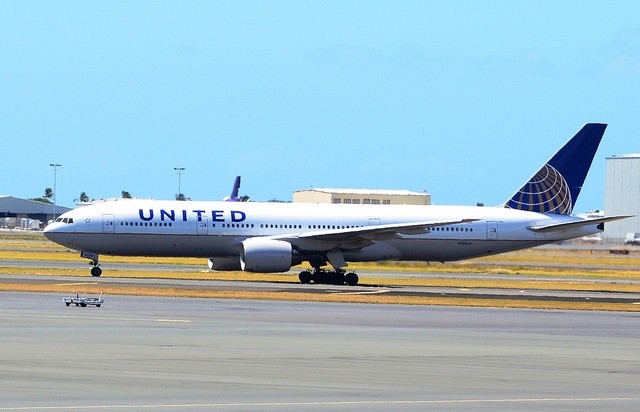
[42,123,637,285]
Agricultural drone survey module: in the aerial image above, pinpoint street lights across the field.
[49,163,61,221]
[174,168,184,195]
